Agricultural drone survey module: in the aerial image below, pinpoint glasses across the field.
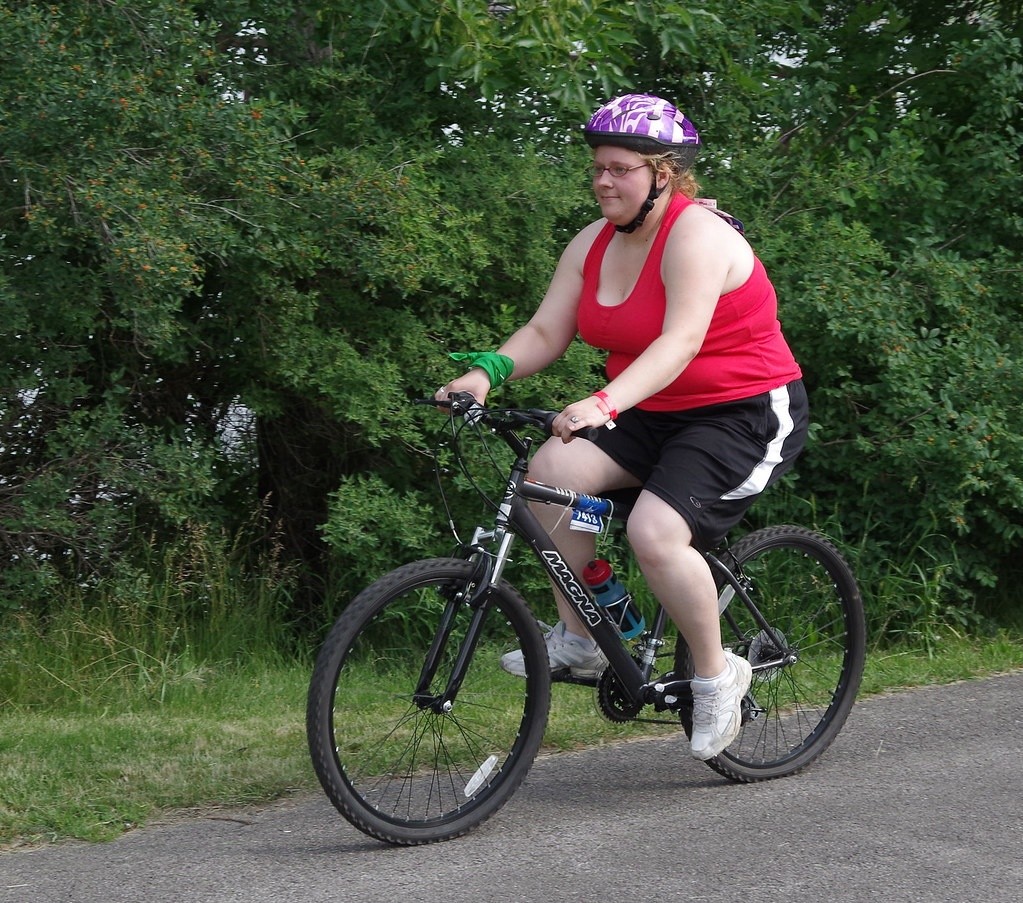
[584,163,650,178]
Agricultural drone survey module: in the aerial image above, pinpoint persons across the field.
[433,93,809,762]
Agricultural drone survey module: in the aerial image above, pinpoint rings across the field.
[440,386,446,393]
[570,417,578,423]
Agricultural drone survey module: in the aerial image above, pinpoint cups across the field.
[584,559,646,639]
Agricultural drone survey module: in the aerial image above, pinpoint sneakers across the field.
[500,621,609,680]
[690,647,753,762]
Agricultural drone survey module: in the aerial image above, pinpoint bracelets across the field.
[449,352,514,390]
[591,390,618,431]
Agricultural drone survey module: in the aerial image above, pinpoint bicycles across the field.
[306,389,867,845]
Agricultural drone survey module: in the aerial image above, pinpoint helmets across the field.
[583,93,702,176]
